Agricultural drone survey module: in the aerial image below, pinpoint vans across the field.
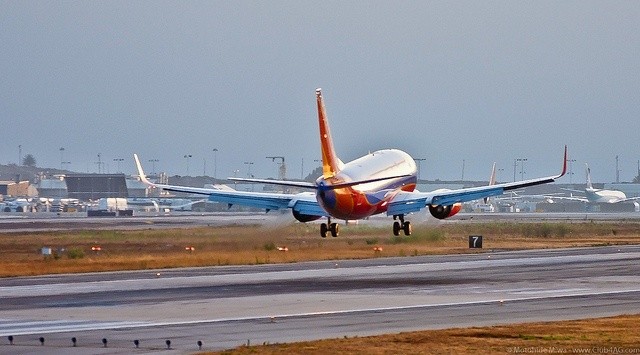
[480,204,494,212]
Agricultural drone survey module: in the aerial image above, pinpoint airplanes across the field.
[544,163,640,203]
[132,88,566,238]
